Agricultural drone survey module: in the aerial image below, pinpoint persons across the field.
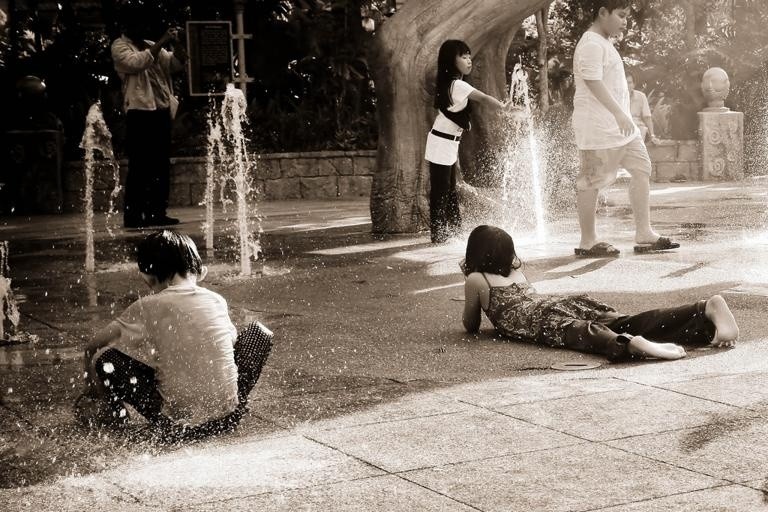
[424,35,530,249]
[571,0,681,259]
[622,72,665,151]
[107,4,197,231]
[70,227,275,443]
[459,225,741,360]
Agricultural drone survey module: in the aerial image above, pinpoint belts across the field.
[430,128,462,141]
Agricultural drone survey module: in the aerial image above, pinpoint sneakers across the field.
[123,216,179,228]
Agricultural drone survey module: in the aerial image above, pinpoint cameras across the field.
[168,23,185,43]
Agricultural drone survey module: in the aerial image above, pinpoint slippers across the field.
[574,242,620,256]
[633,237,680,253]
